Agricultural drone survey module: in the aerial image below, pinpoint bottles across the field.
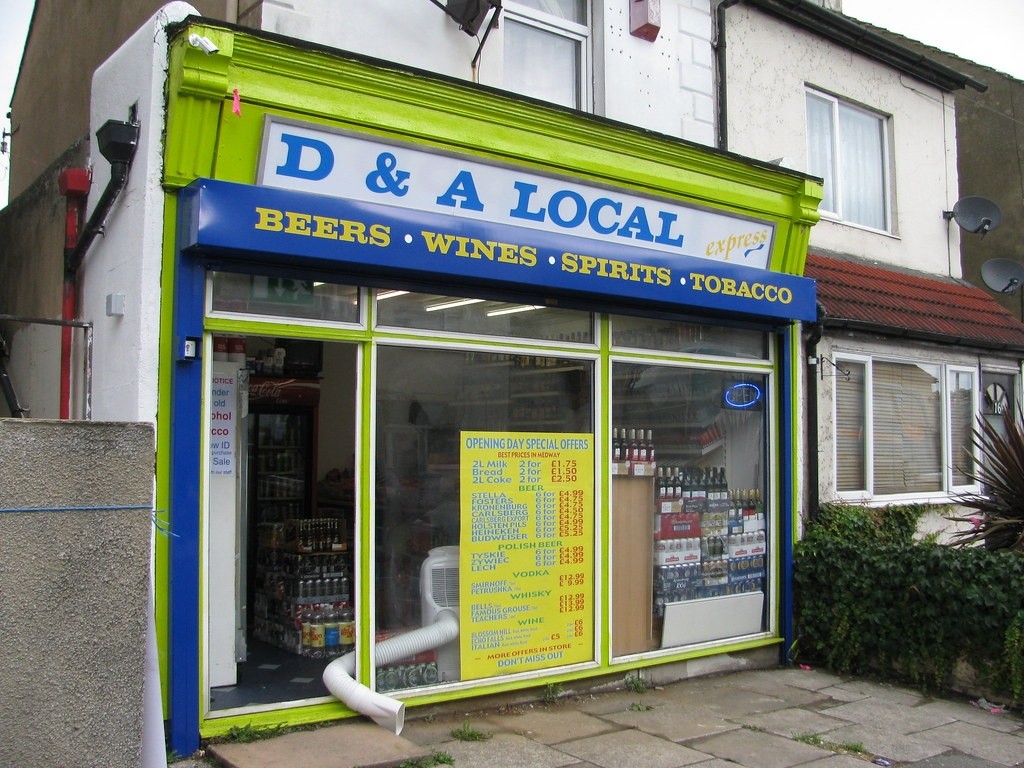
[611,427,655,463]
[653,464,768,618]
[242,412,305,532]
[253,518,357,659]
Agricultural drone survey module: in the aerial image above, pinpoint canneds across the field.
[254,450,303,521]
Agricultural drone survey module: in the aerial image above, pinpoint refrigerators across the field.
[244,376,322,637]
